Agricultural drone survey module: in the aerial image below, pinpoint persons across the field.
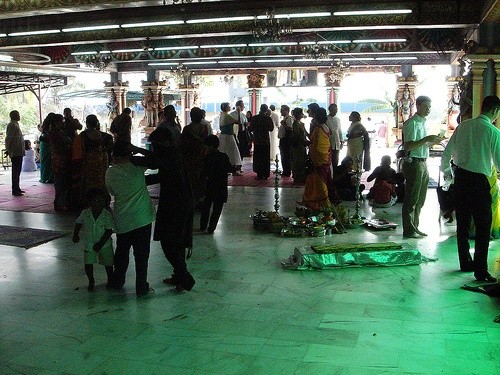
[439,96,500,282]
[72,189,115,290]
[230,101,399,209]
[104,139,153,297]
[403,96,445,238]
[218,103,243,173]
[5,111,27,196]
[130,127,195,292]
[24,108,239,233]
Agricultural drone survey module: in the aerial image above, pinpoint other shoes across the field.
[233,171,243,176]
[460,262,475,272]
[14,193,24,196]
[176,273,195,293]
[163,278,176,284]
[105,279,112,289]
[21,191,25,193]
[416,230,426,236]
[474,269,496,283]
[403,232,423,238]
[87,281,94,290]
[137,287,155,297]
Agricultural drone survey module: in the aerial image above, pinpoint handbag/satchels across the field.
[284,116,293,138]
